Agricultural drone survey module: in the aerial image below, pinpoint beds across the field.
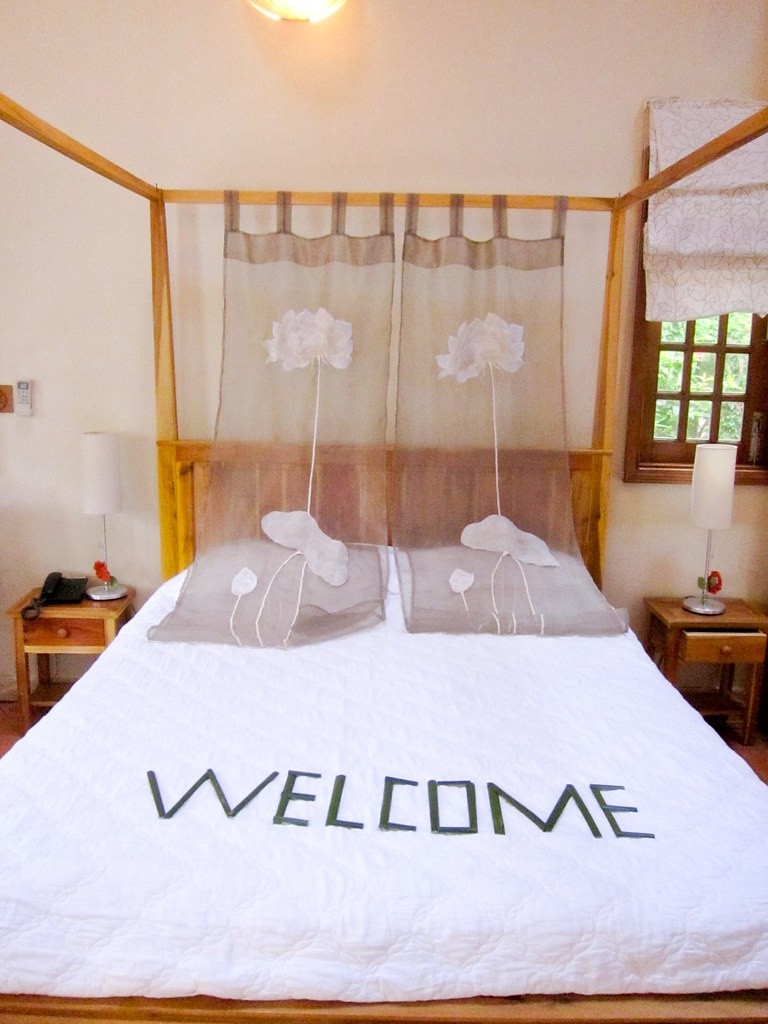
[0,438,768,1024]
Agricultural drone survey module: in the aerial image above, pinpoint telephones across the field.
[40,571,89,605]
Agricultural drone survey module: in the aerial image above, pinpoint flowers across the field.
[697,570,722,593]
[93,560,119,586]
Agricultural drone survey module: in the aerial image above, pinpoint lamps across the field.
[682,444,738,615]
[81,432,127,601]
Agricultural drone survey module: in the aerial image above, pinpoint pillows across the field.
[179,539,609,623]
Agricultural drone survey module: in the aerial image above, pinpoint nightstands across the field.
[5,587,137,736]
[643,597,768,747]
